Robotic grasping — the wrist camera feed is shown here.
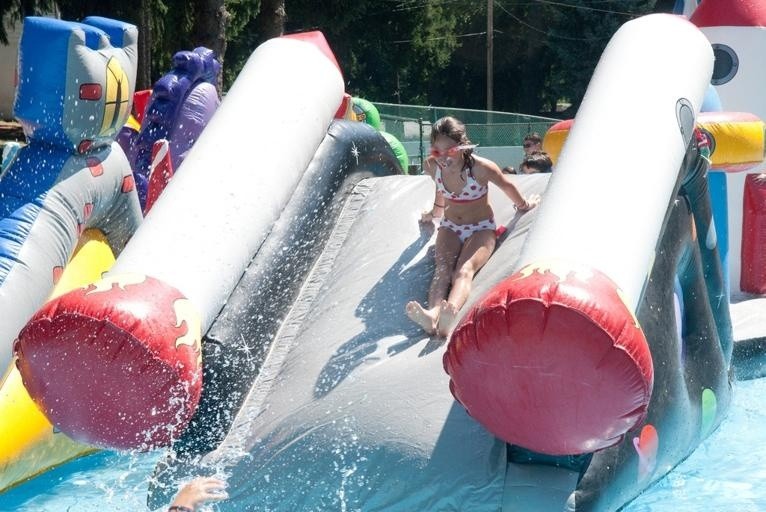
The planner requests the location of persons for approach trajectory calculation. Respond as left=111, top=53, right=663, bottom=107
left=404, top=115, right=540, bottom=339
left=518, top=152, right=553, bottom=174
left=523, top=131, right=542, bottom=154
left=164, top=475, right=228, bottom=512
left=501, top=165, right=517, bottom=174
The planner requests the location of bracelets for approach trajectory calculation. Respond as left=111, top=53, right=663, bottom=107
left=430, top=210, right=441, bottom=219
left=512, top=200, right=526, bottom=207
left=434, top=202, right=444, bottom=209
left=167, top=504, right=196, bottom=512
left=519, top=203, right=529, bottom=211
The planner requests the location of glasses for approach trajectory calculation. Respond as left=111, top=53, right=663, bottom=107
left=523, top=143, right=532, bottom=148
left=428, top=145, right=462, bottom=160
